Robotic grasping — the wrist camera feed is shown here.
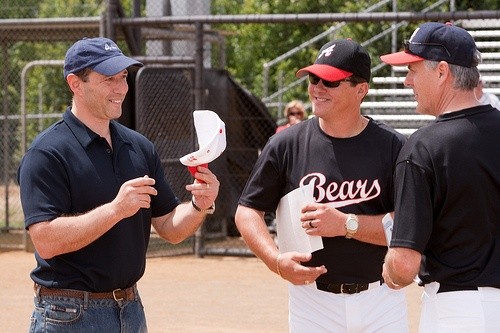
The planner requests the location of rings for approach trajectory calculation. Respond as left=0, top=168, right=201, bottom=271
left=309, top=221, right=314, bottom=228
left=305, top=281, right=310, bottom=285
left=206, top=184, right=210, bottom=189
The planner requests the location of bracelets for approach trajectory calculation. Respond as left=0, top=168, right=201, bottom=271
left=276, top=253, right=283, bottom=279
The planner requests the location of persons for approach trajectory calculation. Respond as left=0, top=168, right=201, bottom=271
left=20, top=37, right=220, bottom=333
left=235, top=40, right=408, bottom=333
left=275, top=100, right=306, bottom=133
left=382, top=22, right=500, bottom=333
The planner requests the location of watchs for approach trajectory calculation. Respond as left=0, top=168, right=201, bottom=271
left=344, top=214, right=359, bottom=238
left=192, top=198, right=215, bottom=214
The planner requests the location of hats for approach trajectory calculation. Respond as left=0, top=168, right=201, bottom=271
left=297, top=37, right=371, bottom=82
left=64, top=37, right=144, bottom=79
left=380, top=23, right=476, bottom=68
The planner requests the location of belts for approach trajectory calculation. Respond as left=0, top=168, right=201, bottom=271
left=34, top=283, right=136, bottom=302
left=316, top=281, right=368, bottom=295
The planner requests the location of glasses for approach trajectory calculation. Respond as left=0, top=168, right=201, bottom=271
left=402, top=39, right=451, bottom=57
left=309, top=72, right=369, bottom=88
left=290, top=112, right=301, bottom=115
